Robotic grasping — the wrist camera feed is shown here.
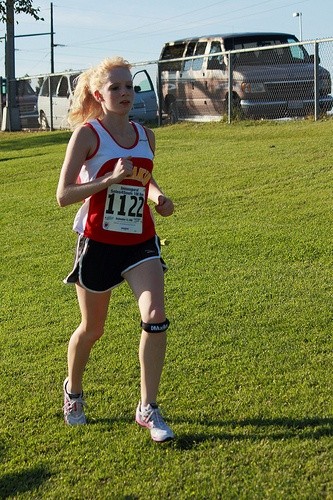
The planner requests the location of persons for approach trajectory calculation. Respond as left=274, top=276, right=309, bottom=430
left=55, top=57, right=178, bottom=442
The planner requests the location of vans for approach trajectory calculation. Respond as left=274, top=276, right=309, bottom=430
left=158, top=33, right=333, bottom=116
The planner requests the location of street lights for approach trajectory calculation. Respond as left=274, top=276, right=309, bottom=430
left=292, top=12, right=304, bottom=60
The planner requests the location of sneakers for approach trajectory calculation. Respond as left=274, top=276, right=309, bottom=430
left=136, top=399, right=175, bottom=443
left=63, top=376, right=88, bottom=426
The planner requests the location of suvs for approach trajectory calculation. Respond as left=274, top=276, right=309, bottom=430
left=36, top=70, right=159, bottom=126
left=0, top=80, right=40, bottom=130
